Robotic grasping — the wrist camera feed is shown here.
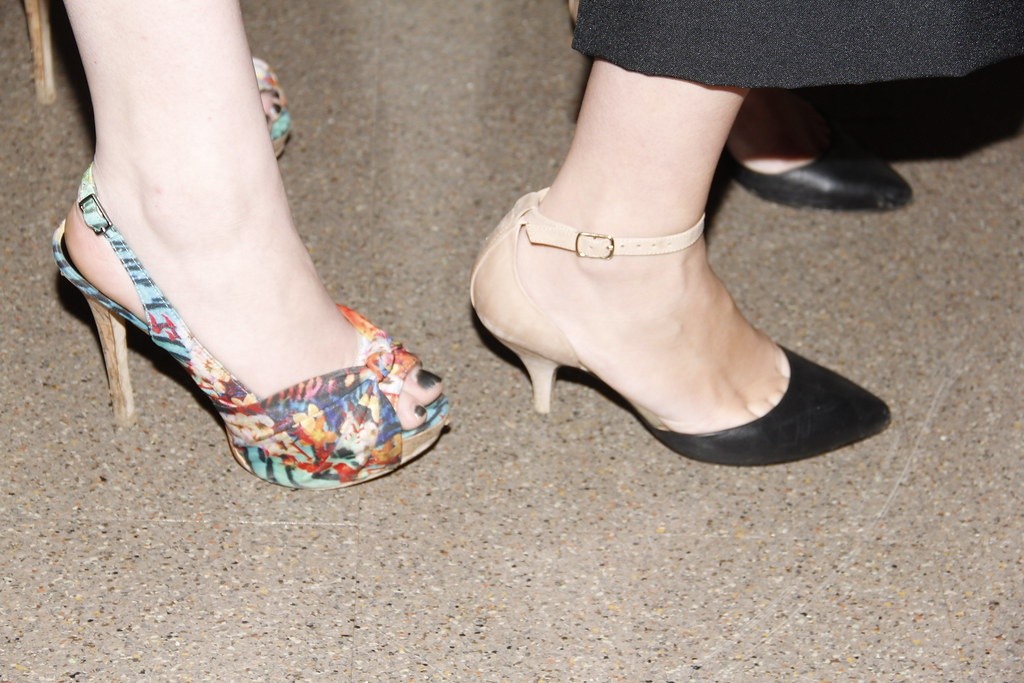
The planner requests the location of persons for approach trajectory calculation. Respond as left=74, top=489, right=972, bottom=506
left=52, top=0, right=449, bottom=491
left=469, top=0, right=1024, bottom=470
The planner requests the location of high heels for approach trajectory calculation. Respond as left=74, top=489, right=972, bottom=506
left=22, top=0, right=291, bottom=159
left=567, top=0, right=912, bottom=211
left=51, top=163, right=451, bottom=490
left=469, top=185, right=892, bottom=467
